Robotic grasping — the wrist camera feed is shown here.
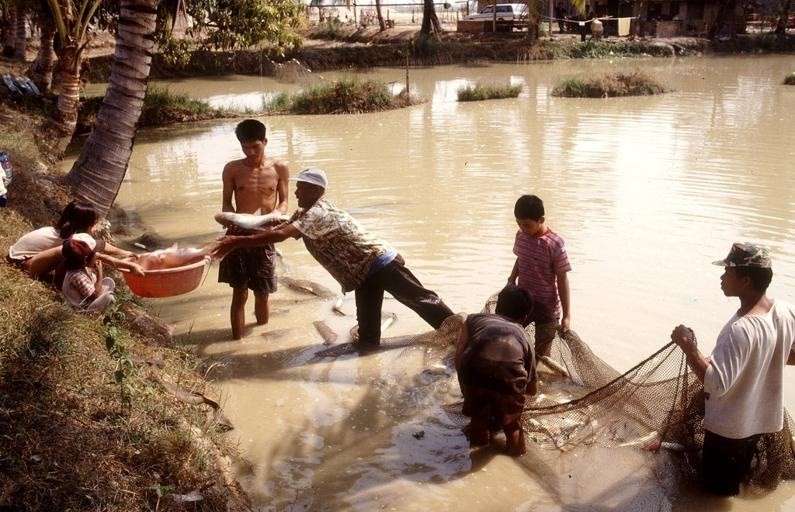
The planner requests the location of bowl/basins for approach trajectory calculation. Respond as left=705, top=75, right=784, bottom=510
left=113, top=246, right=211, bottom=300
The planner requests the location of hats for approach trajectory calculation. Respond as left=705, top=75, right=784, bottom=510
left=61, top=233, right=105, bottom=261
left=712, top=241, right=772, bottom=269
left=288, top=167, right=328, bottom=190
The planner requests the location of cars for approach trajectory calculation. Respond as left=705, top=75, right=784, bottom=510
left=462, top=3, right=534, bottom=27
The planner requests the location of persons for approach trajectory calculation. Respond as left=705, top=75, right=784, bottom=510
left=0, top=163, right=8, bottom=207
left=507, top=195, right=572, bottom=366
left=211, top=168, right=465, bottom=354
left=218, top=119, right=290, bottom=340
left=62, top=232, right=116, bottom=323
left=455, top=286, right=538, bottom=456
left=6, top=199, right=146, bottom=289
left=671, top=242, right=795, bottom=497
